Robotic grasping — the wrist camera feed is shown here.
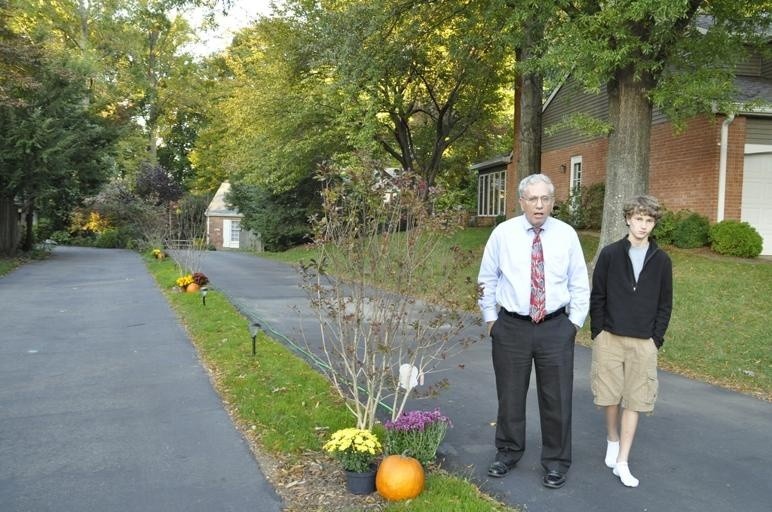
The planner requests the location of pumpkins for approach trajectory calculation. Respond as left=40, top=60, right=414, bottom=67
left=376, top=455, right=425, bottom=503
left=186, top=283, right=200, bottom=293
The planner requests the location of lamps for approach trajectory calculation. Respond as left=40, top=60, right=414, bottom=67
left=559, top=163, right=567, bottom=174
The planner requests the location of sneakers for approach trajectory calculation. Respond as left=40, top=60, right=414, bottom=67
left=613, top=461, right=639, bottom=489
left=604, top=439, right=621, bottom=469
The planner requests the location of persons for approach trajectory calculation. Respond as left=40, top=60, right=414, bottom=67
left=477, top=174, right=592, bottom=489
left=589, top=195, right=673, bottom=487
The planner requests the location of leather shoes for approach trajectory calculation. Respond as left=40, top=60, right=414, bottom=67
left=542, top=469, right=567, bottom=489
left=486, top=459, right=512, bottom=478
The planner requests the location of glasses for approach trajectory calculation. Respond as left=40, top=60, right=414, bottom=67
left=522, top=197, right=554, bottom=206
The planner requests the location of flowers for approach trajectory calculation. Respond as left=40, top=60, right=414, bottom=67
left=322, top=428, right=383, bottom=472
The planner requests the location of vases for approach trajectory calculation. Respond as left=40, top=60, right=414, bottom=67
left=345, top=464, right=377, bottom=494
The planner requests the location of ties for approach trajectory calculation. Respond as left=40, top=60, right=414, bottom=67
left=527, top=228, right=548, bottom=324
